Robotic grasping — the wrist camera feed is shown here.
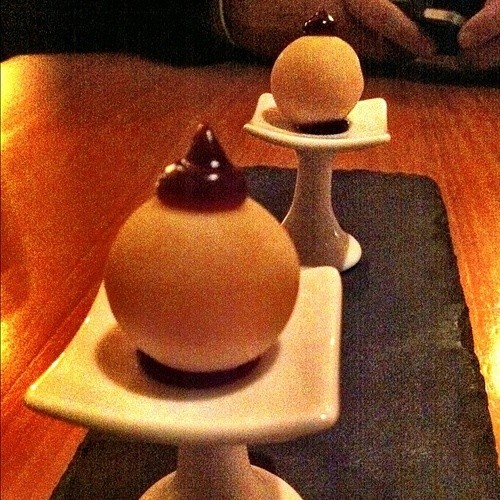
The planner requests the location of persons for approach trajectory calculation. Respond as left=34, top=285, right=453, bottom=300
left=0, top=0, right=500, bottom=82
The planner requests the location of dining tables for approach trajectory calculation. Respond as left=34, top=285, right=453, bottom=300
left=0, top=54, right=500, bottom=500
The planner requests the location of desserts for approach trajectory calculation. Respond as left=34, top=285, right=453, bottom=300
left=271, top=10, right=364, bottom=135
left=103, top=126, right=300, bottom=389
